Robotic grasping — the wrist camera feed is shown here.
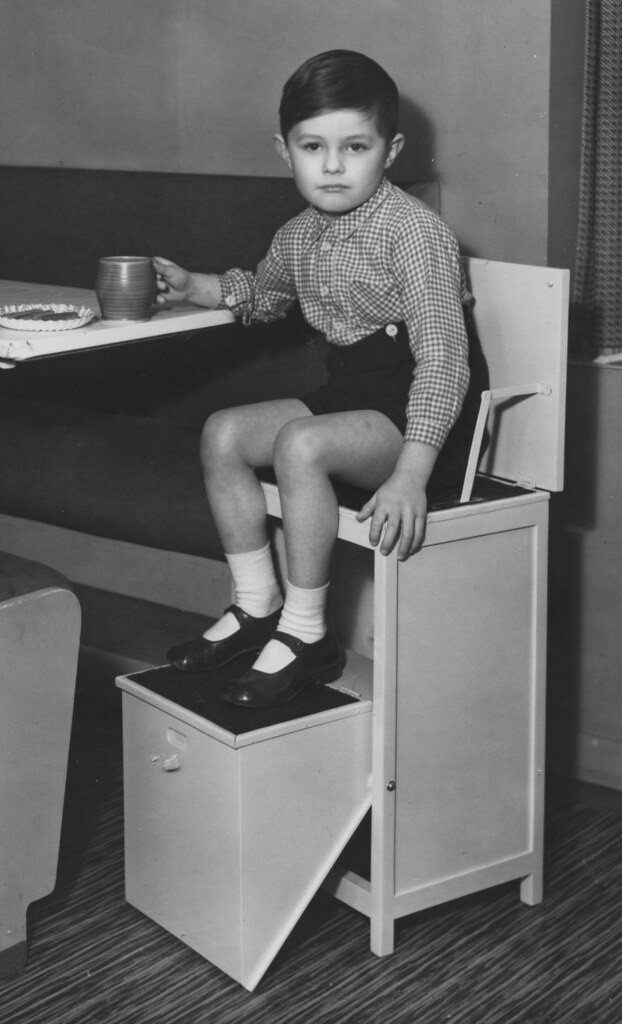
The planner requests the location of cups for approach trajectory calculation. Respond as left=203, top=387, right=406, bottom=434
left=95, top=255, right=156, bottom=323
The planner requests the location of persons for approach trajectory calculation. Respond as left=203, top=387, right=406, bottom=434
left=151, top=50, right=473, bottom=707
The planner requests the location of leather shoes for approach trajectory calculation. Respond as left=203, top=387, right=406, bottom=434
left=220, top=627, right=344, bottom=708
left=166, top=604, right=283, bottom=672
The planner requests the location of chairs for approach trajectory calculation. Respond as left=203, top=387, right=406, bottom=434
left=115, top=257, right=569, bottom=991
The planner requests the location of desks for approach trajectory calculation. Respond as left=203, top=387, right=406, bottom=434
left=0, top=277, right=240, bottom=369
